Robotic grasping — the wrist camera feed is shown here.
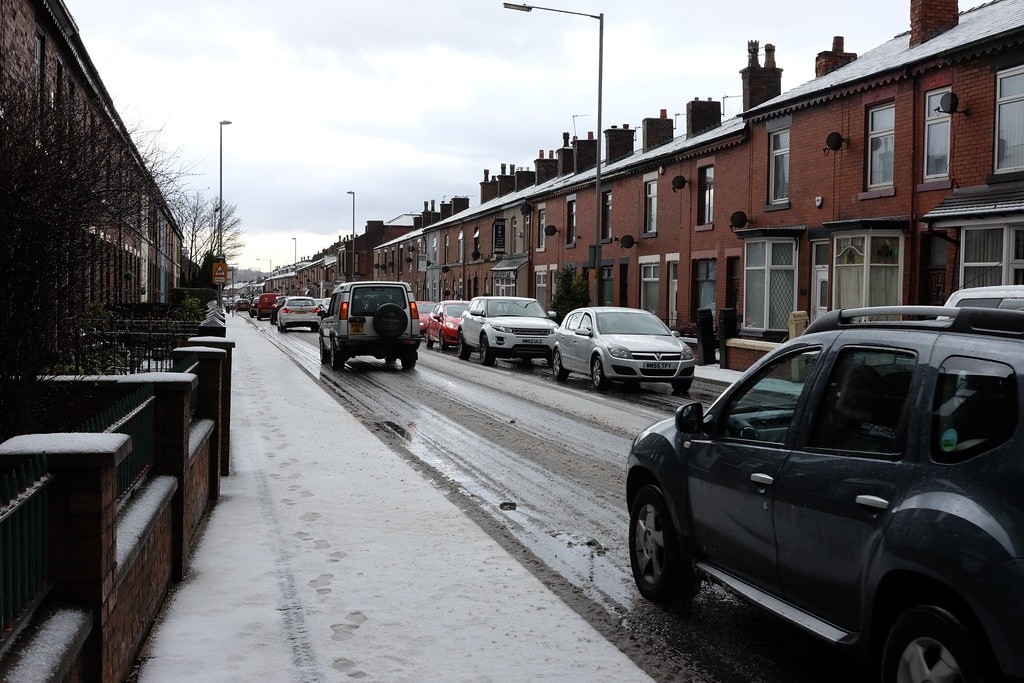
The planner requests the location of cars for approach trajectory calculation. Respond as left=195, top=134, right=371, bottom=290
left=222, top=293, right=332, bottom=325
left=416, top=301, right=438, bottom=333
left=276, top=297, right=321, bottom=334
left=551, top=307, right=696, bottom=394
left=425, top=301, right=471, bottom=351
left=457, top=296, right=559, bottom=366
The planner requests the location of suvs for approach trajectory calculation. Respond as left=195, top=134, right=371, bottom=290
left=317, top=281, right=421, bottom=372
left=623, top=306, right=1024, bottom=681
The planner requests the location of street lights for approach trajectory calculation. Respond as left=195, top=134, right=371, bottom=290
left=220, top=120, right=232, bottom=310
left=502, top=0, right=605, bottom=308
left=347, top=191, right=355, bottom=280
left=292, top=238, right=297, bottom=295
left=256, top=259, right=271, bottom=284
